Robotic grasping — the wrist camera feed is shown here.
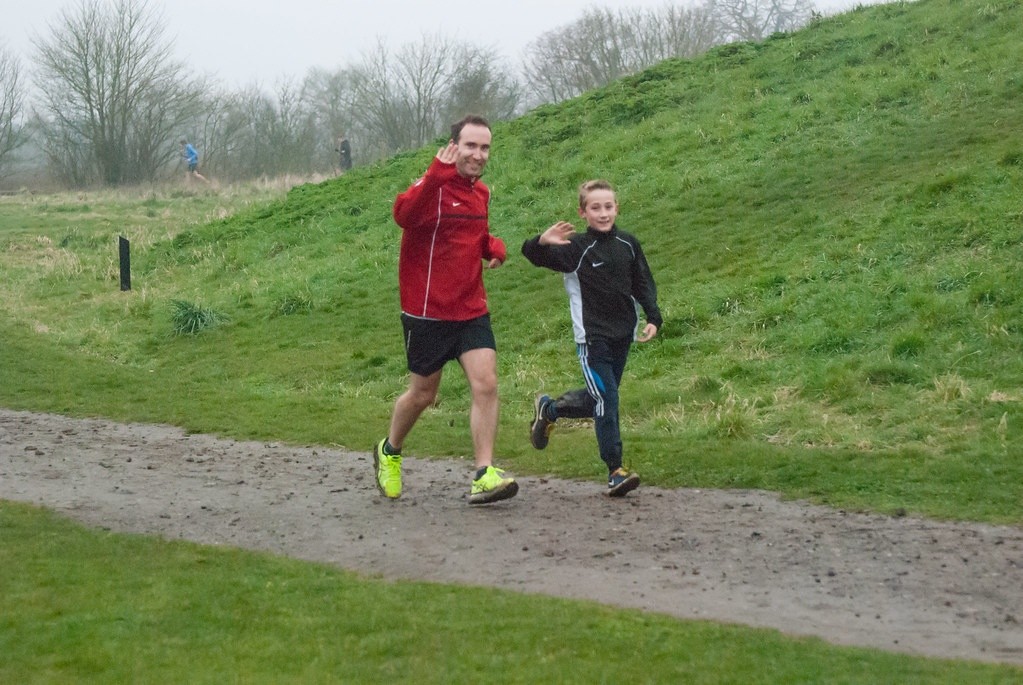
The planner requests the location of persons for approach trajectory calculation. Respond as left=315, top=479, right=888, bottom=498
left=335, top=134, right=352, bottom=172
left=374, top=116, right=518, bottom=504
left=181, top=139, right=211, bottom=189
left=521, top=180, right=661, bottom=497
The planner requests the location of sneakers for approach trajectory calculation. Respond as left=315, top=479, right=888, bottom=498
left=605, top=467, right=641, bottom=498
left=373, top=437, right=403, bottom=499
left=467, top=465, right=519, bottom=504
left=529, top=394, right=555, bottom=450
